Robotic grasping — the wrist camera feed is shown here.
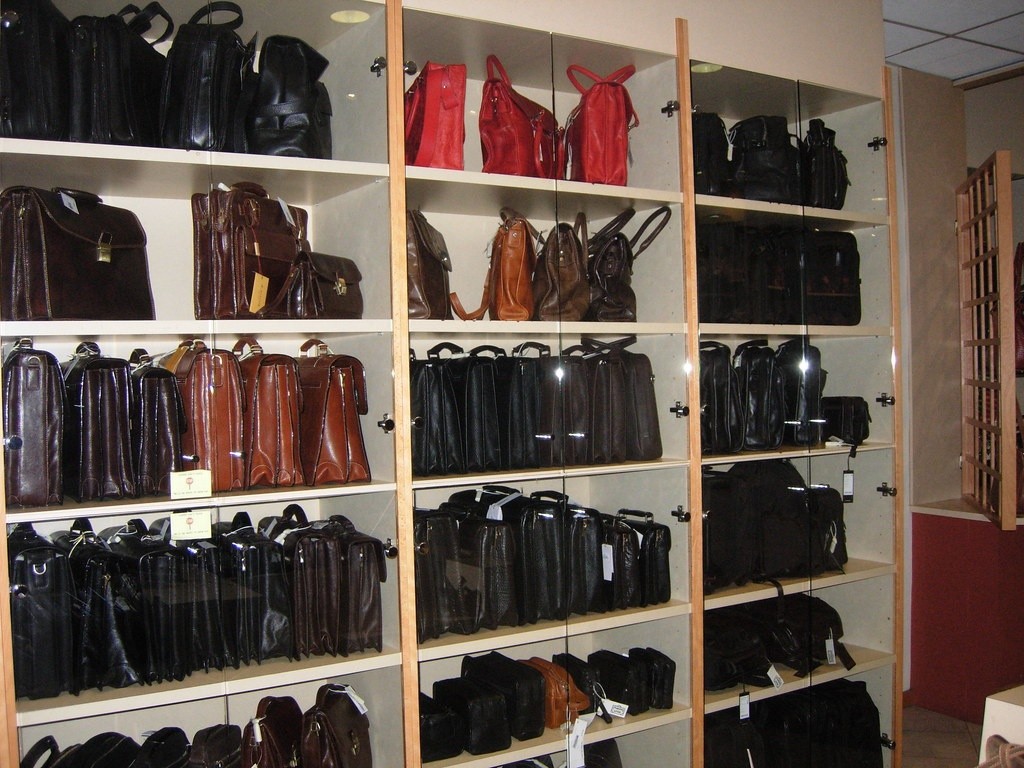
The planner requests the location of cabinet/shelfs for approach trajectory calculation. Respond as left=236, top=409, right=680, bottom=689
left=0, top=0, right=903, bottom=768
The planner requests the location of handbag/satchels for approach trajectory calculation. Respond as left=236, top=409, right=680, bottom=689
left=728, top=115, right=801, bottom=204
left=805, top=118, right=852, bottom=210
left=160, top=2, right=258, bottom=153
left=693, top=112, right=730, bottom=193
left=68, top=1, right=174, bottom=147
left=479, top=55, right=566, bottom=180
left=405, top=61, right=466, bottom=169
left=0, top=0, right=71, bottom=138
left=1, top=184, right=896, bottom=768
left=564, top=65, right=639, bottom=186
left=249, top=34, right=333, bottom=159
